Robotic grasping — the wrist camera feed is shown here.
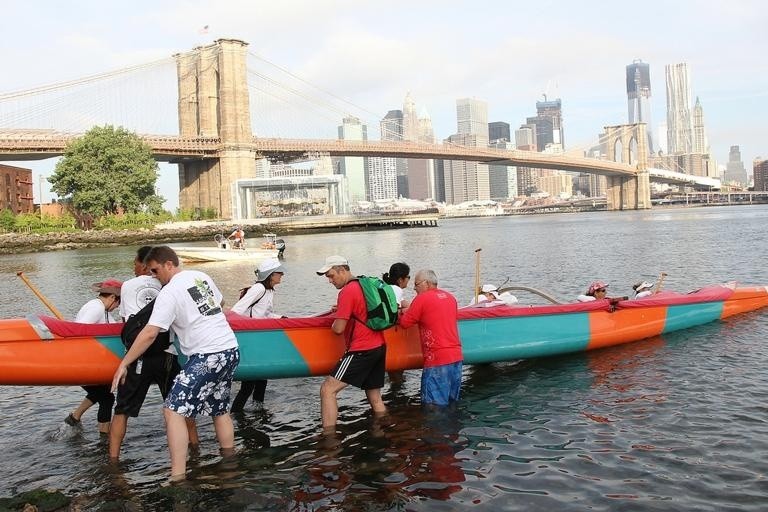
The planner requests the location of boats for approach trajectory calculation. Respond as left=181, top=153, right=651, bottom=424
left=170, top=247, right=280, bottom=261
left=0, top=284, right=768, bottom=385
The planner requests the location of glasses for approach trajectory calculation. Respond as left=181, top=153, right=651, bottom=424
left=598, top=289, right=606, bottom=292
left=414, top=279, right=426, bottom=286
left=148, top=263, right=164, bottom=275
left=405, top=276, right=411, bottom=279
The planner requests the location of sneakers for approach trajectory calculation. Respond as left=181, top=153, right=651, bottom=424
left=64, top=413, right=82, bottom=428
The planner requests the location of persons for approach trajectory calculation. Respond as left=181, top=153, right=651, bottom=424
left=111, top=245, right=242, bottom=492
left=398, top=270, right=464, bottom=405
left=468, top=283, right=499, bottom=311
left=229, top=255, right=289, bottom=412
left=109, top=245, right=199, bottom=457
left=571, top=280, right=610, bottom=304
left=380, top=261, right=411, bottom=388
left=632, top=281, right=656, bottom=301
left=316, top=256, right=388, bottom=428
left=66, top=278, right=123, bottom=436
left=226, top=225, right=245, bottom=249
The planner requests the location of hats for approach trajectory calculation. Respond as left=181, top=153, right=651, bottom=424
left=316, top=256, right=349, bottom=276
left=636, top=281, right=654, bottom=291
left=255, top=257, right=286, bottom=282
left=589, top=281, right=609, bottom=294
left=481, top=284, right=499, bottom=300
left=89, top=279, right=121, bottom=296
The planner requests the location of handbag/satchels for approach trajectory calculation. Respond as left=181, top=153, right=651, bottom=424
left=121, top=296, right=173, bottom=356
left=239, top=287, right=257, bottom=307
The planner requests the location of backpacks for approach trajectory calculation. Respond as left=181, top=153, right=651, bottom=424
left=347, top=275, right=398, bottom=330
